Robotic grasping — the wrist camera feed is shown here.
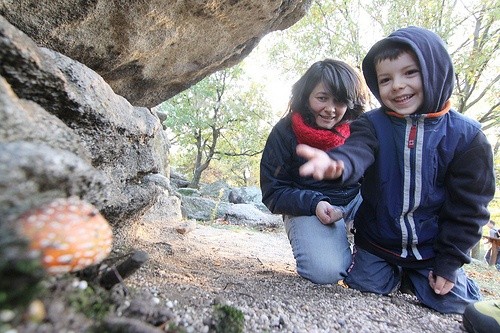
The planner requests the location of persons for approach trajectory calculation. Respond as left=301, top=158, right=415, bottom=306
left=260, top=58, right=365, bottom=284
left=486, top=220, right=500, bottom=272
left=299, top=27, right=496, bottom=315
